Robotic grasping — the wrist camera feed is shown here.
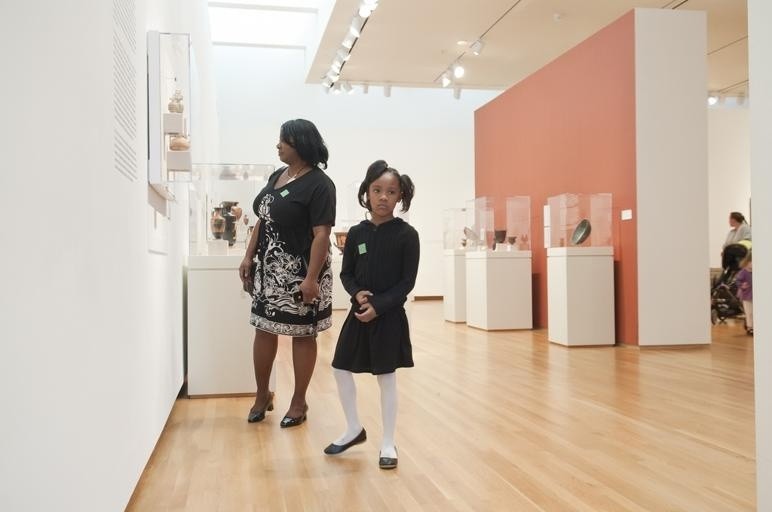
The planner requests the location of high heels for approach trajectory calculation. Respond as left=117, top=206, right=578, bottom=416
left=281, top=403, right=308, bottom=427
left=249, top=391, right=274, bottom=423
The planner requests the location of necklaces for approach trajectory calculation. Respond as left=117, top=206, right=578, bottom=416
left=287, top=166, right=303, bottom=179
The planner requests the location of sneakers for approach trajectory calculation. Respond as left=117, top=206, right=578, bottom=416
left=748, top=328, right=752, bottom=336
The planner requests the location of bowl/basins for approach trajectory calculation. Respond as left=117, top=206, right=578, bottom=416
left=571, top=219, right=591, bottom=244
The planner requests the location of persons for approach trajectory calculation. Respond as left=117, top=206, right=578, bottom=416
left=321, top=160, right=420, bottom=468
left=239, top=118, right=337, bottom=426
left=720, top=211, right=751, bottom=257
left=734, top=251, right=752, bottom=336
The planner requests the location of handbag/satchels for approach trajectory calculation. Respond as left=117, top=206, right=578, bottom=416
left=243, top=262, right=258, bottom=297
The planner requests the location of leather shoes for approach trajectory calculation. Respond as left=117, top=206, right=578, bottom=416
left=380, top=447, right=398, bottom=468
left=324, top=428, right=367, bottom=455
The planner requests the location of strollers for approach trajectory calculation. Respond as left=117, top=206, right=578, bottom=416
left=710, top=239, right=751, bottom=326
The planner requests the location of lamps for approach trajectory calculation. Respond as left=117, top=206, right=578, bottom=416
left=320, top=1, right=393, bottom=98
left=440, top=41, right=484, bottom=99
